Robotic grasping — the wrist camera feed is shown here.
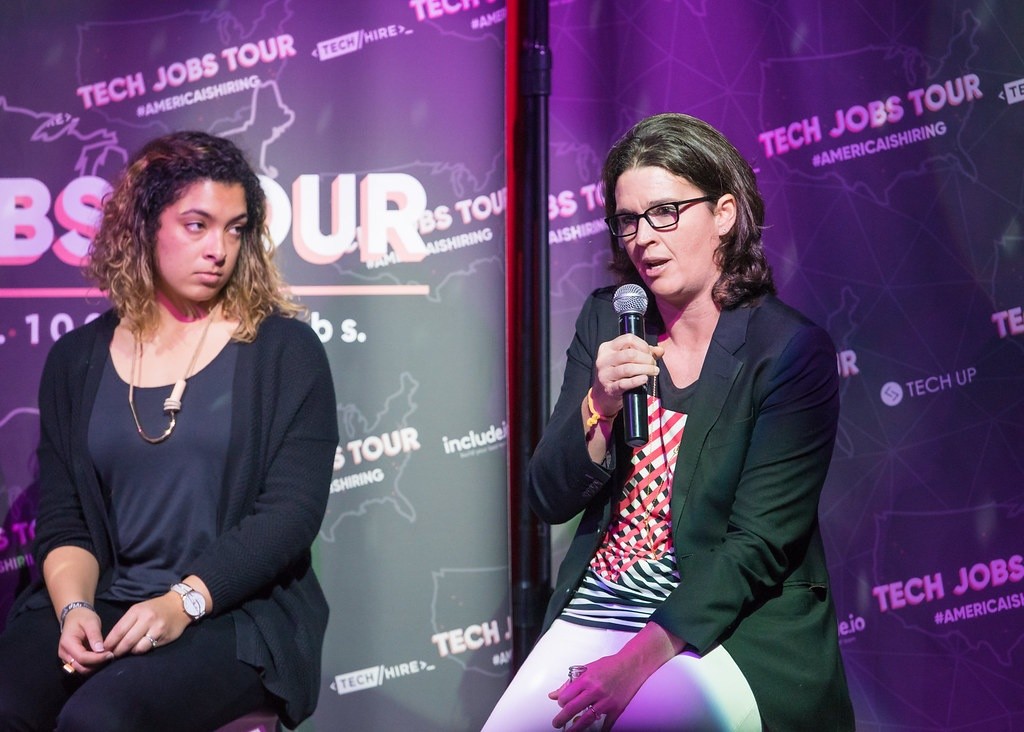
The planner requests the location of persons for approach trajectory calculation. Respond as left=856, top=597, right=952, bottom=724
left=480, top=115, right=857, bottom=732
left=0, top=132, right=338, bottom=732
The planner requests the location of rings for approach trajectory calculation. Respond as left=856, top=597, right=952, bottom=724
left=145, top=633, right=158, bottom=647
left=62, top=658, right=76, bottom=677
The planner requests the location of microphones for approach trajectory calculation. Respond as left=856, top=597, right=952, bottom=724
left=611, top=283, right=649, bottom=448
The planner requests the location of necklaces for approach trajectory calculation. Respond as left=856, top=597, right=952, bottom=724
left=128, top=305, right=219, bottom=443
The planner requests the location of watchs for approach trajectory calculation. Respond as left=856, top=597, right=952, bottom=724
left=170, top=582, right=207, bottom=624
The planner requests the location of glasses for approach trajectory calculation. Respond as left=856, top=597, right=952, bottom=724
left=604, top=195, right=714, bottom=238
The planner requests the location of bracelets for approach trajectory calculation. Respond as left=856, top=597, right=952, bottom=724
left=59, top=601, right=95, bottom=634
left=590, top=707, right=601, bottom=720
left=586, top=387, right=618, bottom=427
